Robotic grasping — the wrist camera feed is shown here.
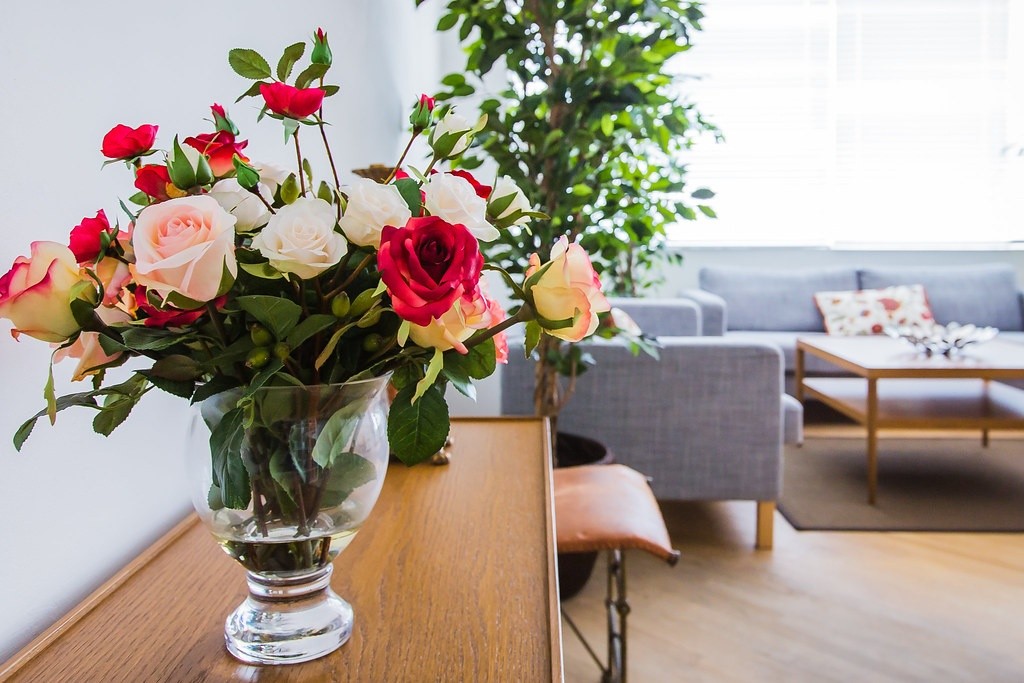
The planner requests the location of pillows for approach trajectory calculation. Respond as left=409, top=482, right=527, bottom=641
left=814, top=284, right=939, bottom=331
left=861, top=263, right=1019, bottom=330
left=700, top=266, right=859, bottom=332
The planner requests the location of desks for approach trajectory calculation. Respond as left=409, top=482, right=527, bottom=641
left=0, top=413, right=565, bottom=683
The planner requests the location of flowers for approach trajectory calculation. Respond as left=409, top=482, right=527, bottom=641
left=0, top=26, right=611, bottom=569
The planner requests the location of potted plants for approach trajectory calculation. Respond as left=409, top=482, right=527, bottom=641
left=399, top=0, right=726, bottom=608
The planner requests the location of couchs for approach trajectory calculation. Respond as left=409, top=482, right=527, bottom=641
left=507, top=245, right=1024, bottom=551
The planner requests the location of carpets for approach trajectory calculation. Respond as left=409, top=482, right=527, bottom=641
left=780, top=435, right=1024, bottom=533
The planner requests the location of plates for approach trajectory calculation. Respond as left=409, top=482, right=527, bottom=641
left=885, top=321, right=999, bottom=355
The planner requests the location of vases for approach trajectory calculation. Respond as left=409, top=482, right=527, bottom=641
left=173, top=370, right=394, bottom=666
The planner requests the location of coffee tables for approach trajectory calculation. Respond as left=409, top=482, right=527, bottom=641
left=795, top=331, right=1024, bottom=505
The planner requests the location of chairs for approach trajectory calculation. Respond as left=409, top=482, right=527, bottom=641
left=553, top=466, right=685, bottom=683
